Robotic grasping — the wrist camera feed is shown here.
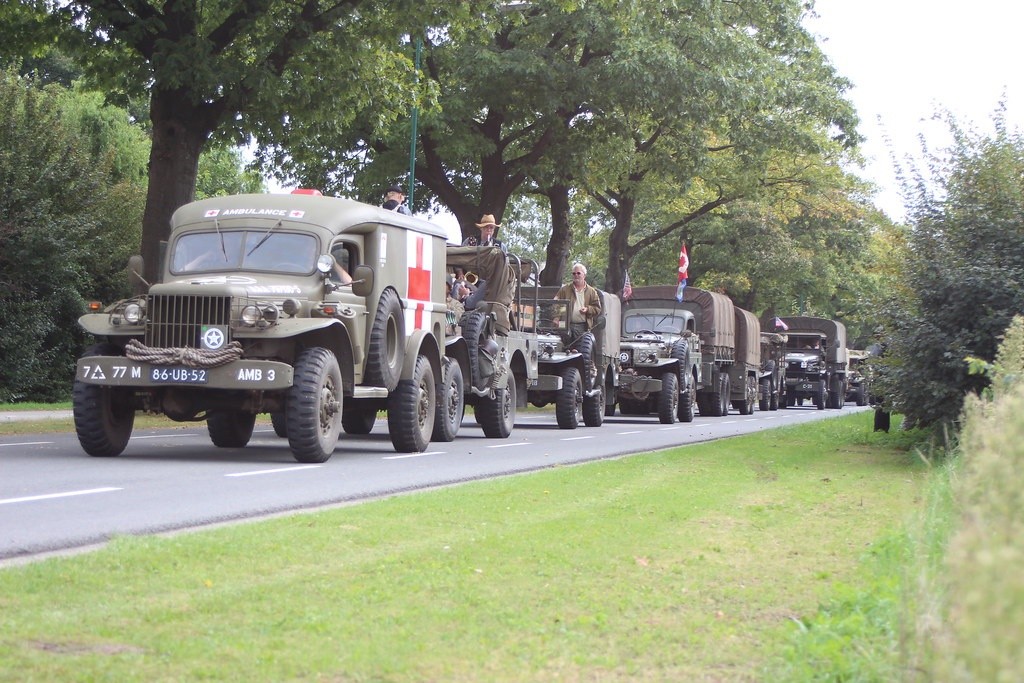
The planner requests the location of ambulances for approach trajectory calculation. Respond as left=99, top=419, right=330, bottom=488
left=73, top=189, right=451, bottom=465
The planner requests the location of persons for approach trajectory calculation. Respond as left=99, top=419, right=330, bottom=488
left=379, top=185, right=412, bottom=216
left=445, top=273, right=465, bottom=335
left=462, top=214, right=509, bottom=264
left=553, top=263, right=601, bottom=345
left=804, top=340, right=819, bottom=349
left=329, top=255, right=352, bottom=286
left=178, top=236, right=236, bottom=271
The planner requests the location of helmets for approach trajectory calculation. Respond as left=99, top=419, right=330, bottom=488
left=446, top=272, right=452, bottom=291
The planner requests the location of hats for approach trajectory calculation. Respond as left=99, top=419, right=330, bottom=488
left=384, top=186, right=402, bottom=196
left=475, top=214, right=502, bottom=228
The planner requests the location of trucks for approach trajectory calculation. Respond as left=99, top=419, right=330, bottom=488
left=434, top=242, right=540, bottom=443
left=504, top=283, right=623, bottom=430
left=758, top=332, right=790, bottom=411
left=812, top=349, right=871, bottom=406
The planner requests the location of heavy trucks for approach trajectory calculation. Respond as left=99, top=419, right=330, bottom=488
left=765, top=315, right=849, bottom=411
left=603, top=284, right=760, bottom=425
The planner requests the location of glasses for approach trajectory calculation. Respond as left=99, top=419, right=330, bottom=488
left=572, top=272, right=581, bottom=275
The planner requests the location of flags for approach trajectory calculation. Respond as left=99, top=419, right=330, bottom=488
left=776, top=317, right=788, bottom=330
left=673, top=278, right=686, bottom=302
left=677, top=241, right=690, bottom=278
left=622, top=270, right=631, bottom=301
